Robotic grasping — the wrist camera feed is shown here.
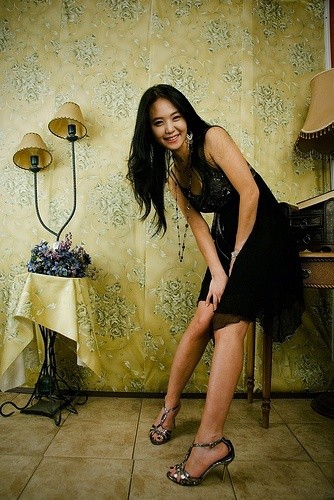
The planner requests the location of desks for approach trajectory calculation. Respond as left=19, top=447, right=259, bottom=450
left=244, top=251, right=334, bottom=428
left=0, top=271, right=102, bottom=425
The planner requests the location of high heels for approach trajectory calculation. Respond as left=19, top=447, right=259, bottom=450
left=167, top=435, right=235, bottom=486
left=150, top=401, right=182, bottom=445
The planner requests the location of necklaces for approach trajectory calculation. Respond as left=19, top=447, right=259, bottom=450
left=167, top=140, right=192, bottom=263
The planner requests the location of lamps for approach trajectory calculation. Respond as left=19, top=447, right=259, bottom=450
left=294, top=68, right=334, bottom=163
left=13, top=102, right=87, bottom=242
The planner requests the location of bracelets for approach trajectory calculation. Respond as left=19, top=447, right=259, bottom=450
left=231, top=251, right=239, bottom=257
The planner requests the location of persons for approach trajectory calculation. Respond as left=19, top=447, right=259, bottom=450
left=126, top=82, right=306, bottom=486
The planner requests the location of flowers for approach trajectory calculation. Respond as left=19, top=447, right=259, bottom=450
left=27, top=232, right=99, bottom=278
